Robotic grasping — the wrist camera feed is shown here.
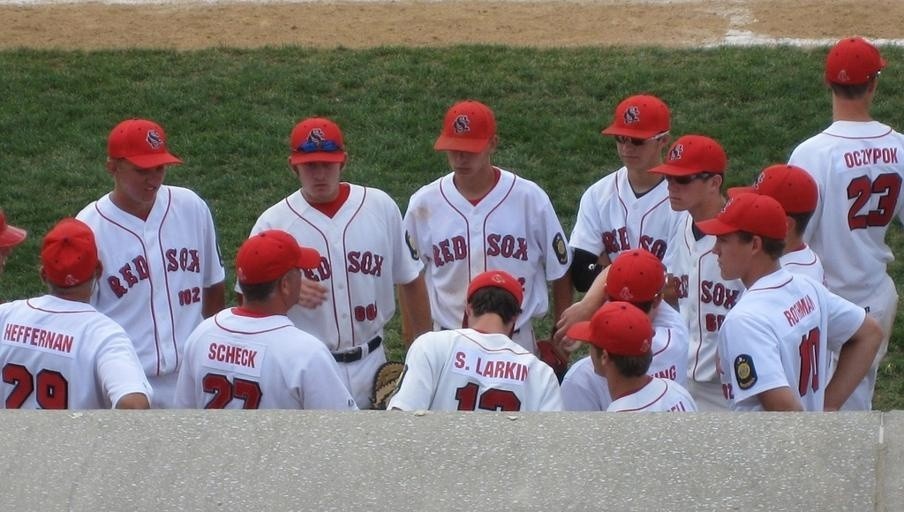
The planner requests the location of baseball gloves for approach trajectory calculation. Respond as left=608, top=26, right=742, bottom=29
left=372, top=361, right=405, bottom=410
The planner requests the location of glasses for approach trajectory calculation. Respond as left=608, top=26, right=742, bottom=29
left=661, top=171, right=712, bottom=186
left=612, top=128, right=665, bottom=147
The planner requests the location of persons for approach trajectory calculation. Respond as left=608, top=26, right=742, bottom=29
left=76, top=118, right=226, bottom=409
left=695, top=193, right=883, bottom=411
left=788, top=37, right=904, bottom=410
left=174, top=231, right=357, bottom=409
left=0, top=217, right=154, bottom=409
left=0, top=211, right=27, bottom=275
left=566, top=301, right=697, bottom=412
left=397, top=100, right=572, bottom=385
left=554, top=250, right=690, bottom=411
left=727, top=164, right=825, bottom=284
left=233, top=115, right=432, bottom=409
left=386, top=272, right=564, bottom=412
left=646, top=133, right=745, bottom=412
left=568, top=94, right=687, bottom=327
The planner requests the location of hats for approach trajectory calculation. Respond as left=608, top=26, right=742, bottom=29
left=692, top=190, right=789, bottom=243
left=107, top=118, right=183, bottom=173
left=430, top=99, right=499, bottom=156
left=607, top=247, right=670, bottom=308
left=599, top=91, right=673, bottom=141
left=38, top=218, right=100, bottom=288
left=236, top=230, right=321, bottom=282
left=647, top=132, right=728, bottom=179
left=1, top=210, right=27, bottom=250
left=288, top=116, right=350, bottom=171
left=459, top=270, right=524, bottom=337
left=568, top=298, right=656, bottom=357
left=823, top=34, right=886, bottom=90
left=728, top=165, right=821, bottom=216
